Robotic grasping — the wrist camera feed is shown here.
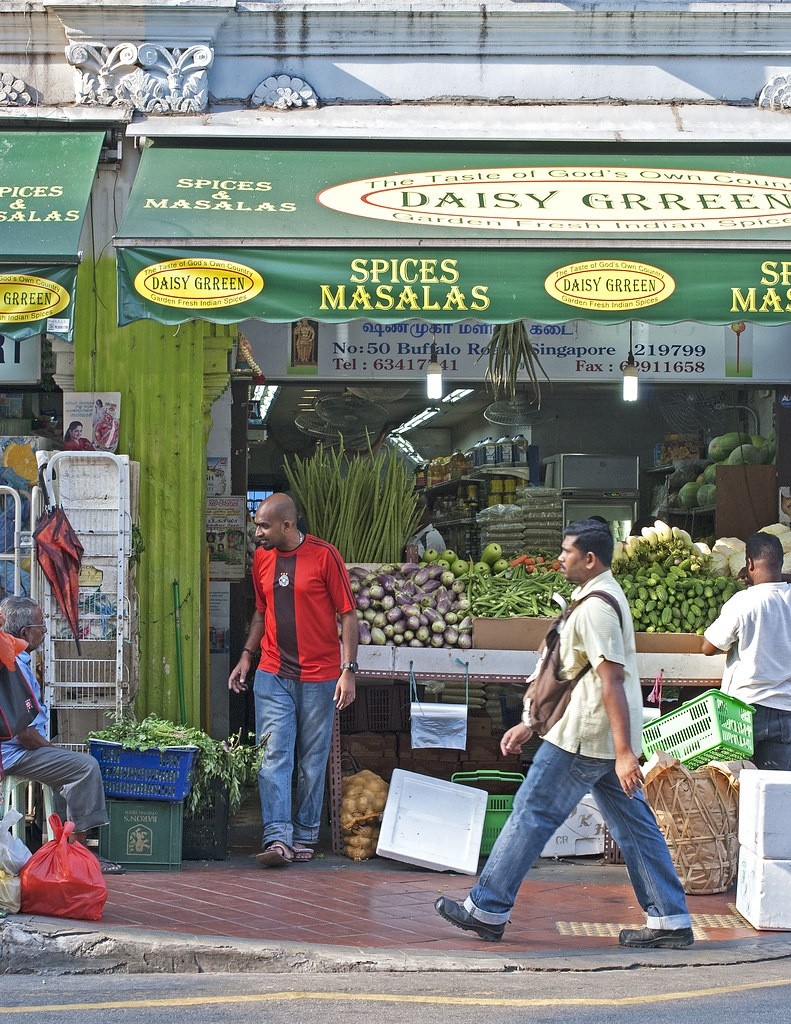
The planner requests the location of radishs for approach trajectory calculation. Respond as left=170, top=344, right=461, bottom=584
left=612, top=521, right=712, bottom=562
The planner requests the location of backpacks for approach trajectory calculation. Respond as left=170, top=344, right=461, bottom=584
left=522, top=590, right=622, bottom=737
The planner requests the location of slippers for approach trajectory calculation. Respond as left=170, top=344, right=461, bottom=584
left=256, top=845, right=293, bottom=864
left=292, top=846, right=315, bottom=861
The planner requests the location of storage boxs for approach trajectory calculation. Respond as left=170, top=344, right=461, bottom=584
left=89, top=739, right=231, bottom=870
left=341, top=614, right=791, bottom=933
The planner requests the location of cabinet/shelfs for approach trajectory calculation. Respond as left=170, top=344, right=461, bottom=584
left=415, top=461, right=532, bottom=558
left=47, top=449, right=140, bottom=846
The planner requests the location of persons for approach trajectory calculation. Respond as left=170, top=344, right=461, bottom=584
left=64, top=421, right=96, bottom=451
left=702, top=531, right=791, bottom=771
left=0, top=595, right=127, bottom=875
left=435, top=515, right=694, bottom=950
left=228, top=493, right=358, bottom=867
left=94, top=399, right=119, bottom=451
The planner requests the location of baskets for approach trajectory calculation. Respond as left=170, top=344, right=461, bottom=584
left=452, top=769, right=526, bottom=856
left=640, top=688, right=756, bottom=770
left=640, top=770, right=739, bottom=895
left=90, top=741, right=200, bottom=800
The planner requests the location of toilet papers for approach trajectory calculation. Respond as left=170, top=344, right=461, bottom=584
left=643, top=707, right=661, bottom=723
left=608, top=520, right=619, bottom=538
left=618, top=520, right=632, bottom=541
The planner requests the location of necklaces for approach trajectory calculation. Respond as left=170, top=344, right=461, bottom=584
left=297, top=530, right=303, bottom=544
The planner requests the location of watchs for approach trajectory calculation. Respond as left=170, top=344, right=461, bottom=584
left=343, top=660, right=358, bottom=673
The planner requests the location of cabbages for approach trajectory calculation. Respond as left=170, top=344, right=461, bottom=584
left=694, top=523, right=791, bottom=579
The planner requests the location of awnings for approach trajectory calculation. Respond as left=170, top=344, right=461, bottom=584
left=0, top=132, right=106, bottom=346
left=109, top=149, right=791, bottom=327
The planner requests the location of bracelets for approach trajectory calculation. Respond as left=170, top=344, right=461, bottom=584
left=240, top=648, right=256, bottom=658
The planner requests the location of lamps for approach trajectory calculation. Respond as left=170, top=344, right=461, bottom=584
left=387, top=389, right=475, bottom=464
left=248, top=386, right=282, bottom=426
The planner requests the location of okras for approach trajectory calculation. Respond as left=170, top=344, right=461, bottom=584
left=457, top=546, right=579, bottom=619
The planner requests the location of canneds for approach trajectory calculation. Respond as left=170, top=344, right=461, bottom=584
left=457, top=474, right=529, bottom=507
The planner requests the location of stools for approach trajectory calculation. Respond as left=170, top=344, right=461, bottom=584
left=1, top=776, right=58, bottom=853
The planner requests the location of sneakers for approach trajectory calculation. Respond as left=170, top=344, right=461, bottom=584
left=435, top=897, right=506, bottom=941
left=619, top=927, right=694, bottom=948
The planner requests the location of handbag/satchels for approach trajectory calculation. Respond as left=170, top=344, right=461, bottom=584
left=0, top=659, right=41, bottom=742
left=0, top=870, right=21, bottom=915
left=18, top=813, right=107, bottom=921
left=0, top=807, right=33, bottom=876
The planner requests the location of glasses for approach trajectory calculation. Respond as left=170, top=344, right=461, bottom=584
left=25, top=621, right=46, bottom=629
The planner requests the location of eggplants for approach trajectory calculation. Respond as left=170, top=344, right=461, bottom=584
left=347, top=559, right=472, bottom=648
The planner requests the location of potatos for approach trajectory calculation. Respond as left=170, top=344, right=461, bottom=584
left=340, top=770, right=388, bottom=860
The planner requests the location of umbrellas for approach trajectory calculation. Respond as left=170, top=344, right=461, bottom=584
left=32, top=461, right=86, bottom=656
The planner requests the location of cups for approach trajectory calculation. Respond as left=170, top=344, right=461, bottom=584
left=93, top=420, right=119, bottom=454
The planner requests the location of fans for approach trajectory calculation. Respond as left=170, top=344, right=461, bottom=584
left=656, top=386, right=759, bottom=448
left=483, top=383, right=557, bottom=427
left=294, top=382, right=412, bottom=443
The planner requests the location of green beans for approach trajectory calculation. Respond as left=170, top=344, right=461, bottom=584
left=85, top=710, right=271, bottom=818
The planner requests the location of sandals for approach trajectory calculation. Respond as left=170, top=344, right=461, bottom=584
left=91, top=851, right=126, bottom=874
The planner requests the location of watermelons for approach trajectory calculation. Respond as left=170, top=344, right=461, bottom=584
left=668, top=432, right=776, bottom=509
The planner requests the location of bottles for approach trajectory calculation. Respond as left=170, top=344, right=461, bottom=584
left=512, top=434, right=529, bottom=467
left=494, top=435, right=514, bottom=468
left=444, top=449, right=466, bottom=482
left=464, top=441, right=485, bottom=471
left=483, top=437, right=496, bottom=468
left=416, top=456, right=449, bottom=486
left=429, top=485, right=487, bottom=521
